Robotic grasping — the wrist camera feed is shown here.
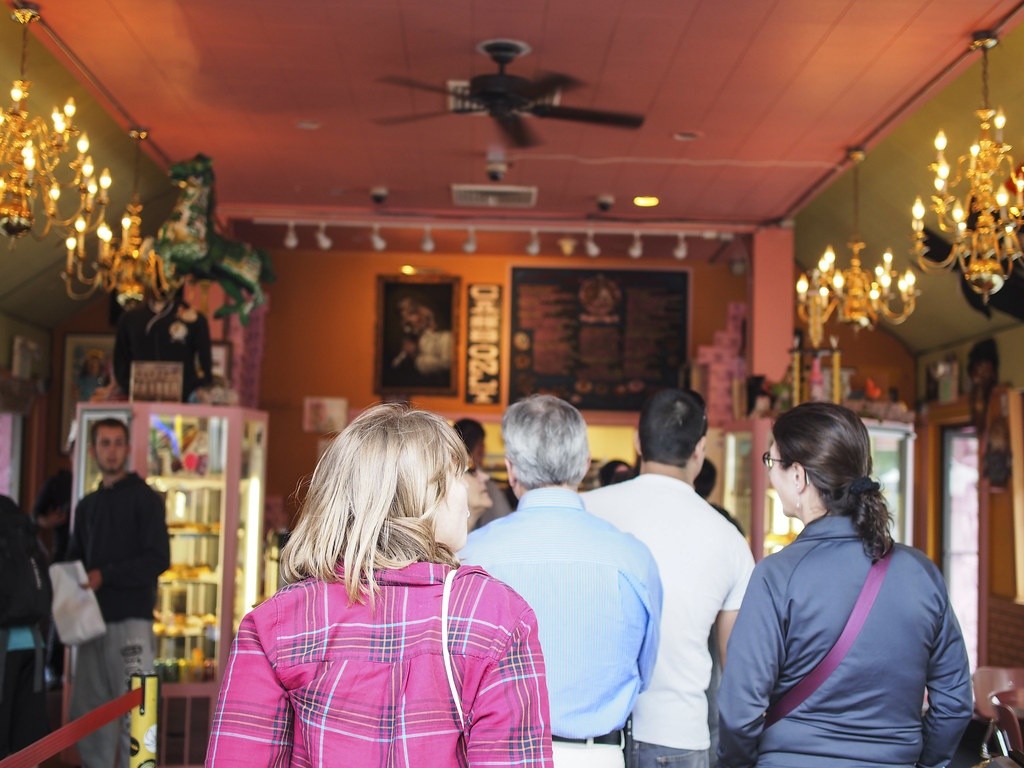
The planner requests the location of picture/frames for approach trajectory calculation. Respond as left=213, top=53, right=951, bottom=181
left=59, top=332, right=126, bottom=446
left=371, top=272, right=462, bottom=393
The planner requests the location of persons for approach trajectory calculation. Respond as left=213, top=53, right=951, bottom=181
left=452, top=395, right=663, bottom=767
left=0, top=494, right=34, bottom=750
left=454, top=418, right=512, bottom=534
left=578, top=386, right=757, bottom=768
left=66, top=416, right=171, bottom=767
left=204, top=402, right=553, bottom=768
left=599, top=457, right=746, bottom=536
left=34, top=470, right=72, bottom=689
left=717, top=402, right=974, bottom=768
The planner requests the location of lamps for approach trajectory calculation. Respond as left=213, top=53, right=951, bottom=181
left=797, top=145, right=922, bottom=348
left=907, top=27, right=1024, bottom=302
left=0, top=0, right=196, bottom=304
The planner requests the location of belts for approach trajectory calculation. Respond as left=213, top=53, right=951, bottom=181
left=550, top=729, right=622, bottom=745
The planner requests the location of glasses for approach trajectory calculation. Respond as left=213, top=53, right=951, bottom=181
left=762, top=451, right=811, bottom=485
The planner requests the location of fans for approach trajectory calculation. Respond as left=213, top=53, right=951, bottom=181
left=368, top=38, right=646, bottom=147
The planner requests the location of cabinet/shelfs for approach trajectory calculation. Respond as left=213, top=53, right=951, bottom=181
left=65, top=403, right=270, bottom=680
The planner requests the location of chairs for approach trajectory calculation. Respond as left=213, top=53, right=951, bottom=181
left=160, top=682, right=220, bottom=768
